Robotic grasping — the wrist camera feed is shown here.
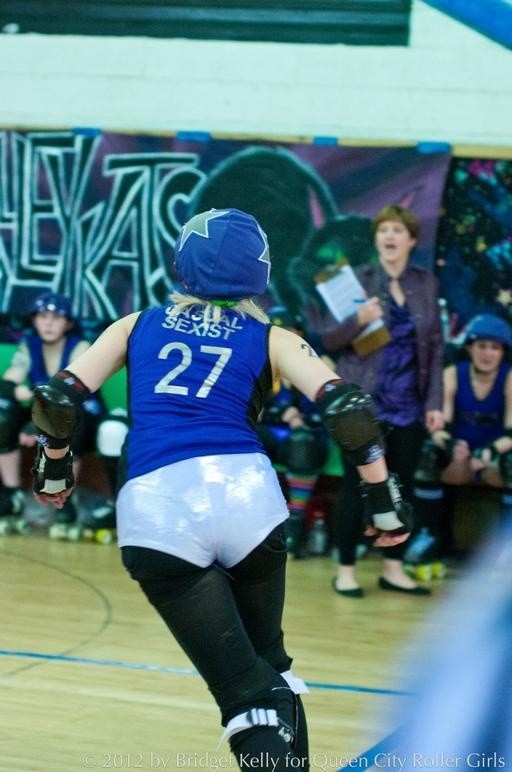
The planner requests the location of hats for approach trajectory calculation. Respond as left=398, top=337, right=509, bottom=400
left=174, top=208, right=271, bottom=300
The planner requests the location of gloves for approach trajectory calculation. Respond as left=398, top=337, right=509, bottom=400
left=287, top=430, right=325, bottom=472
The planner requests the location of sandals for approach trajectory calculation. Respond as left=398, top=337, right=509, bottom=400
left=378, top=577, right=431, bottom=595
left=332, top=577, right=363, bottom=597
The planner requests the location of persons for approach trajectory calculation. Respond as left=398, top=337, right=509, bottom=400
left=1, top=203, right=512, bottom=597
left=31, top=209, right=414, bottom=772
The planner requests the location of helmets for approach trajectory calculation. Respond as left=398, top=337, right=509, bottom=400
left=29, top=292, right=71, bottom=318
left=462, top=313, right=512, bottom=348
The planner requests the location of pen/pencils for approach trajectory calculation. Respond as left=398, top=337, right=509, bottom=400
left=353, top=298, right=368, bottom=303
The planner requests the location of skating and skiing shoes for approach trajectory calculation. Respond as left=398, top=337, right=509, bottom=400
left=0, top=488, right=118, bottom=544
left=402, top=498, right=458, bottom=585
